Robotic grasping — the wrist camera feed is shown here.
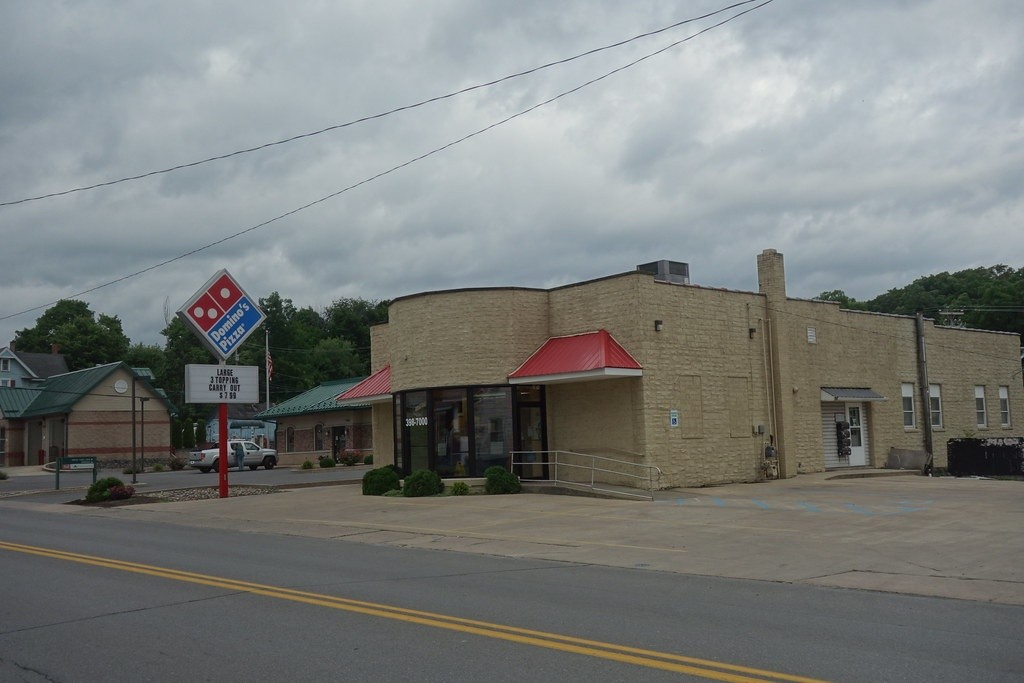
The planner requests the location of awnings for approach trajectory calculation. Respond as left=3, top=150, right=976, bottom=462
left=821, top=387, right=889, bottom=402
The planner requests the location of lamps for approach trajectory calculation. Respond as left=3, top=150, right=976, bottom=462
left=345, top=427, right=349, bottom=434
left=325, top=430, right=329, bottom=436
left=43, top=434, right=46, bottom=440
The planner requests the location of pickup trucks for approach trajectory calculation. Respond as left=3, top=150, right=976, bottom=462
left=188, top=438, right=280, bottom=472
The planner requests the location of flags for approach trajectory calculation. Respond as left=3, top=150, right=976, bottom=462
left=265, top=349, right=273, bottom=380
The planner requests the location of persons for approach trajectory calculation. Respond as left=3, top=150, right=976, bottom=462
left=338, top=434, right=345, bottom=463
